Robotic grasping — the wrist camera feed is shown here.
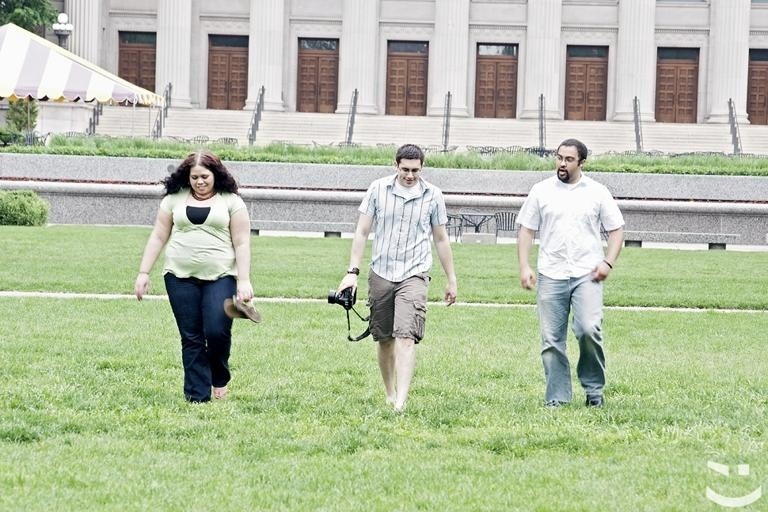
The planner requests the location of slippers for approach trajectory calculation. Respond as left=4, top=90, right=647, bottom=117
left=221, top=293, right=262, bottom=325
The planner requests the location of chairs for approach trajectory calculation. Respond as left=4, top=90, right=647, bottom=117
left=0, top=131, right=240, bottom=148
left=267, top=139, right=768, bottom=159
left=446, top=207, right=521, bottom=242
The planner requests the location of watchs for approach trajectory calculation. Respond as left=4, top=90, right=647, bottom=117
left=345, top=266, right=360, bottom=276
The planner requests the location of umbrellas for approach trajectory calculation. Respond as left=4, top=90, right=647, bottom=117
left=0, top=21, right=169, bottom=116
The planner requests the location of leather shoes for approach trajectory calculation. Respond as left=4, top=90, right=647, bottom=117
left=546, top=396, right=604, bottom=408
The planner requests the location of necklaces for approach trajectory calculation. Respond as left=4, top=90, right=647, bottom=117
left=188, top=188, right=218, bottom=201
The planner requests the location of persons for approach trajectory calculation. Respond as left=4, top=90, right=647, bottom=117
left=516, top=138, right=626, bottom=412
left=333, top=143, right=459, bottom=417
left=133, top=150, right=256, bottom=404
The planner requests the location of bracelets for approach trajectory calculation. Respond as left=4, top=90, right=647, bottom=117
left=602, top=259, right=613, bottom=270
left=137, top=270, right=149, bottom=275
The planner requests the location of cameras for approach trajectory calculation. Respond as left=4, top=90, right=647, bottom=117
left=328, top=288, right=356, bottom=310
